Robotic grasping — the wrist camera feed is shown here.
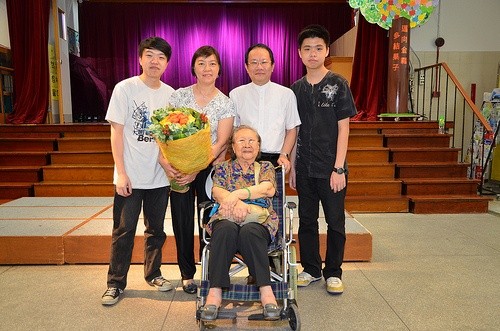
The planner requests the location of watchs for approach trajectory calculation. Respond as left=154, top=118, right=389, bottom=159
left=333, top=167, right=345, bottom=174
left=281, top=153, right=288, bottom=158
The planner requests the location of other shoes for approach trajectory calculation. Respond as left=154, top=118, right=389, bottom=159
left=263, top=303, right=280, bottom=321
left=182, top=279, right=198, bottom=293
left=200, top=302, right=222, bottom=322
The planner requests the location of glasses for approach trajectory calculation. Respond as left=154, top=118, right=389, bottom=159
left=248, top=60, right=273, bottom=68
left=235, top=138, right=261, bottom=144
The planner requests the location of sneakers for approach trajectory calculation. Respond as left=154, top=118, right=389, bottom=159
left=148, top=276, right=173, bottom=291
left=297, top=271, right=322, bottom=287
left=101, top=287, right=123, bottom=305
left=326, top=277, right=344, bottom=293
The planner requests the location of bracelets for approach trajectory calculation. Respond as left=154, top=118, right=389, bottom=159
left=244, top=187, right=251, bottom=199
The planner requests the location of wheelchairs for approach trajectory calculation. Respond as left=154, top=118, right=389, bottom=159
left=195, top=162, right=303, bottom=331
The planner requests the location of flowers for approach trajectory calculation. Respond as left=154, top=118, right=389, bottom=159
left=149, top=107, right=210, bottom=147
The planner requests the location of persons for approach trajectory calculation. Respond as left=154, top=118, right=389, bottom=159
left=158, top=46, right=236, bottom=294
left=200, top=125, right=281, bottom=322
left=100, top=37, right=176, bottom=304
left=228, top=43, right=301, bottom=285
left=288, top=27, right=357, bottom=293
